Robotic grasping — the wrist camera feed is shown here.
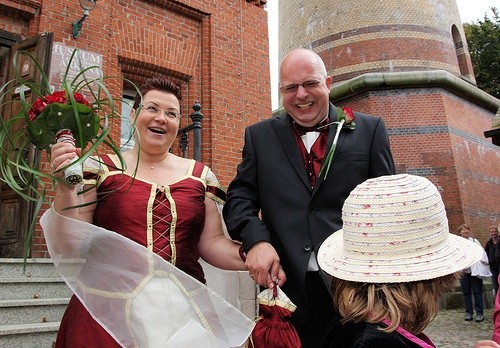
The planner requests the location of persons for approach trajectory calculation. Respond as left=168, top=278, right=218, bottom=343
left=317, top=172, right=486, bottom=348
left=484, top=225, right=500, bottom=306
left=471, top=270, right=500, bottom=348
left=221, top=47, right=396, bottom=348
left=49, top=78, right=286, bottom=348
left=456, top=224, right=485, bottom=322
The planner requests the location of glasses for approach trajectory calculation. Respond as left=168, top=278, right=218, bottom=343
left=134, top=104, right=183, bottom=122
left=281, top=78, right=325, bottom=94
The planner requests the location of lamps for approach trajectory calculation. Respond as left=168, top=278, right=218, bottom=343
left=72, top=0, right=97, bottom=39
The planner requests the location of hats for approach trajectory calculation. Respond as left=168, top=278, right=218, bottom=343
left=317, top=174, right=484, bottom=283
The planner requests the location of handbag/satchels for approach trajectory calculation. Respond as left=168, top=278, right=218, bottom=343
left=239, top=244, right=302, bottom=348
left=482, top=265, right=493, bottom=284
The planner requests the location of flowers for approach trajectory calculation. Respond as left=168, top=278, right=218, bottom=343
left=317, top=106, right=357, bottom=180
left=0, top=48, right=143, bottom=275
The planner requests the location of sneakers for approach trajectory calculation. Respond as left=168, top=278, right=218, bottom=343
left=476, top=314, right=484, bottom=322
left=465, top=314, right=473, bottom=321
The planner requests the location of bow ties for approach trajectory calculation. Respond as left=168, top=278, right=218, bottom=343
left=292, top=117, right=330, bottom=137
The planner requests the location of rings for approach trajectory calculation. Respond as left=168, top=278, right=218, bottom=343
left=248, top=273, right=255, bottom=277
left=51, top=162, right=56, bottom=168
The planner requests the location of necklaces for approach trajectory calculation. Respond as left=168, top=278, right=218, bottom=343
left=131, top=150, right=170, bottom=169
